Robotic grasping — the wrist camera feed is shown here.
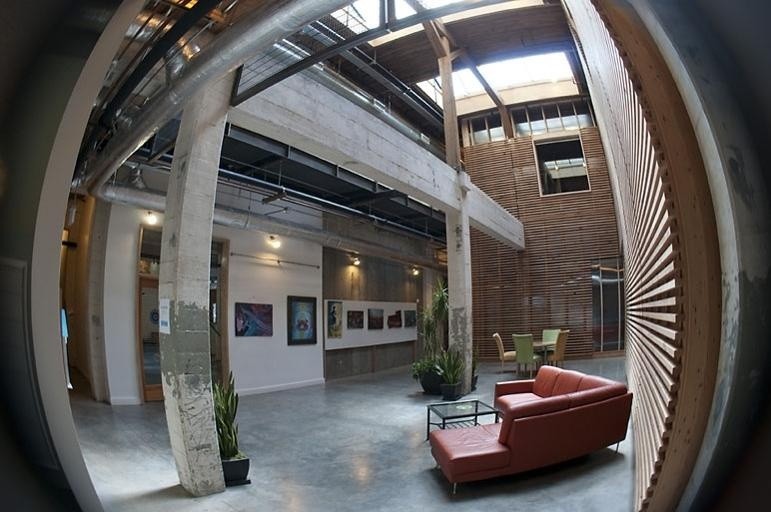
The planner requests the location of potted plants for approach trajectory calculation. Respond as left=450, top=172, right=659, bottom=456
left=407, top=352, right=465, bottom=401
left=211, top=371, right=252, bottom=488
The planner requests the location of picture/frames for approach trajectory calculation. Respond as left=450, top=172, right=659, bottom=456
left=286, top=295, right=317, bottom=345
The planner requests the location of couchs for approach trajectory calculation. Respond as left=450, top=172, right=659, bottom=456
left=427, top=364, right=634, bottom=495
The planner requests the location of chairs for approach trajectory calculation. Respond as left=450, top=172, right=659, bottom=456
left=492, top=328, right=571, bottom=380
